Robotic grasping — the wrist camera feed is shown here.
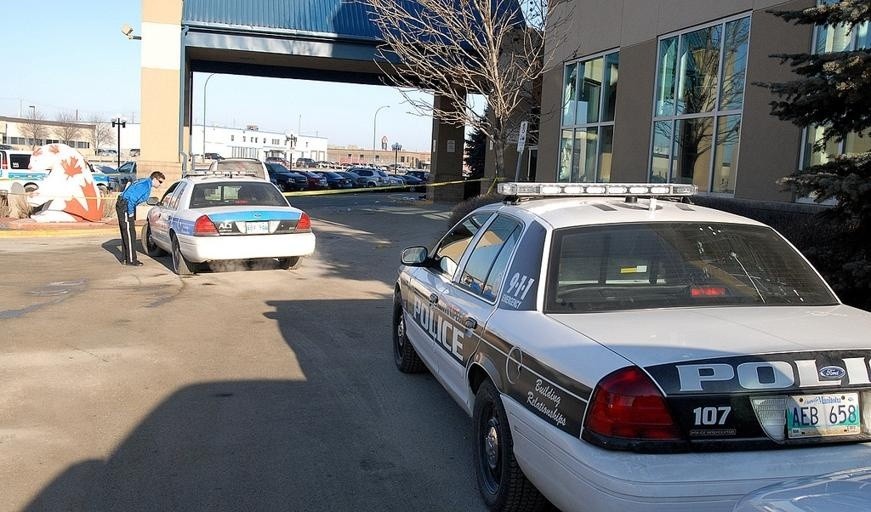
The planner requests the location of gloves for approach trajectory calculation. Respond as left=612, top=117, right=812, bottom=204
left=129, top=214, right=134, bottom=222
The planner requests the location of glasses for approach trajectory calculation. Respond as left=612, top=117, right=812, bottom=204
left=157, top=179, right=162, bottom=184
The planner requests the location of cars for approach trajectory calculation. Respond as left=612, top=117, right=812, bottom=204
left=137, top=173, right=314, bottom=273
left=391, top=164, right=430, bottom=185
left=388, top=181, right=871, bottom=510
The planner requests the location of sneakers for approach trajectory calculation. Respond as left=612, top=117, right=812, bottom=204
left=128, top=261, right=143, bottom=266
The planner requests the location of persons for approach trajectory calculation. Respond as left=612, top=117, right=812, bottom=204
left=114, top=169, right=165, bottom=268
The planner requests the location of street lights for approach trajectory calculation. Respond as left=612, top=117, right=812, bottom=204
left=730, top=252, right=766, bottom=304
left=285, top=130, right=297, bottom=170
left=392, top=142, right=402, bottom=174
left=29, top=104, right=37, bottom=145
left=372, top=105, right=390, bottom=160
left=111, top=115, right=127, bottom=167
left=203, top=73, right=222, bottom=159
